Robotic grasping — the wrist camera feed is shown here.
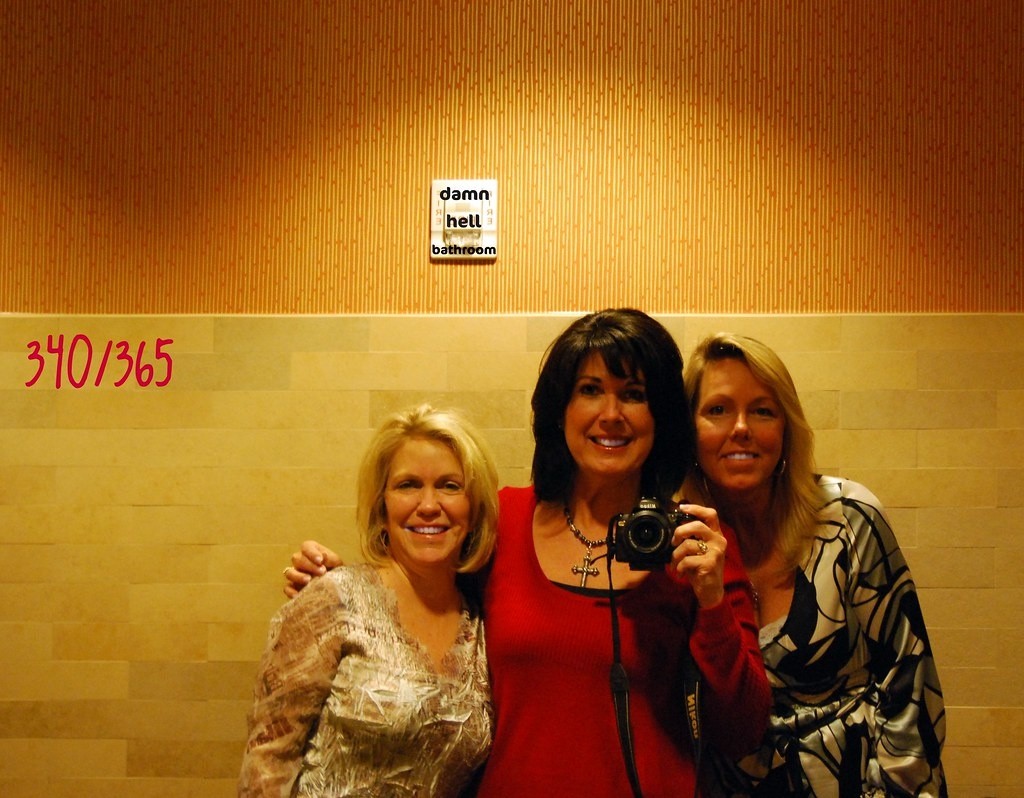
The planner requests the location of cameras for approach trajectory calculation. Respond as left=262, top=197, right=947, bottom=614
left=615, top=497, right=702, bottom=571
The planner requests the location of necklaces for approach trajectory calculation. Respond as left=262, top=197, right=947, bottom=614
left=561, top=498, right=649, bottom=593
left=716, top=500, right=781, bottom=612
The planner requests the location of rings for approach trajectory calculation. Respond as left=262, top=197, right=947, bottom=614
left=692, top=538, right=711, bottom=557
left=282, top=566, right=296, bottom=579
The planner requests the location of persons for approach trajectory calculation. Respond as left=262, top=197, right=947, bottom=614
left=279, top=307, right=771, bottom=798
left=237, top=404, right=500, bottom=798
left=683, top=329, right=948, bottom=798
